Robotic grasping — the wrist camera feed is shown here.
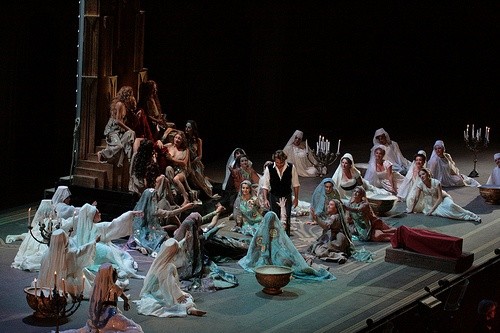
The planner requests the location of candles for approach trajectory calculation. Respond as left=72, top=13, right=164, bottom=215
left=82, top=274, right=86, bottom=291
left=317, top=135, right=330, bottom=154
left=485, top=126, right=490, bottom=142
left=472, top=124, right=474, bottom=138
left=28, top=208, right=32, bottom=226
left=464, top=124, right=470, bottom=139
left=62, top=278, right=65, bottom=293
left=34, top=277, right=37, bottom=297
left=477, top=127, right=481, bottom=140
left=338, top=139, right=342, bottom=153
left=54, top=269, right=57, bottom=288
left=306, top=139, right=308, bottom=153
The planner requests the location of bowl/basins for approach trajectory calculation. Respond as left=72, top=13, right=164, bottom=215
left=479, top=185, right=500, bottom=204
left=366, top=196, right=396, bottom=217
left=253, top=265, right=294, bottom=294
left=24, top=287, right=67, bottom=318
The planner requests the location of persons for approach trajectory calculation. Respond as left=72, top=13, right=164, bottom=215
left=49, top=263, right=146, bottom=333
left=309, top=128, right=482, bottom=265
left=98, top=79, right=239, bottom=290
left=7, top=186, right=146, bottom=301
left=486, top=153, right=500, bottom=186
left=132, top=230, right=208, bottom=319
left=283, top=130, right=327, bottom=178
left=237, top=196, right=336, bottom=282
left=222, top=147, right=311, bottom=240
